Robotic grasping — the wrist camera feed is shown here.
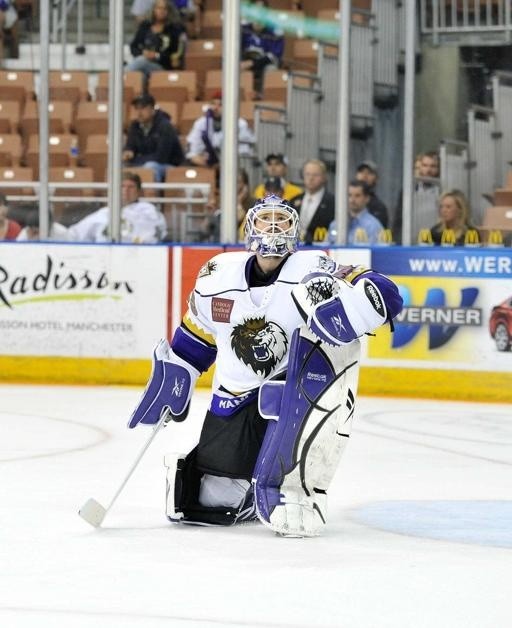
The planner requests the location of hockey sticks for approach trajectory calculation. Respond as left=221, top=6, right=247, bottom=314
left=77, top=407, right=170, bottom=527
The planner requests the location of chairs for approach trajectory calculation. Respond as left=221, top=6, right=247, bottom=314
left=1, top=0, right=512, bottom=246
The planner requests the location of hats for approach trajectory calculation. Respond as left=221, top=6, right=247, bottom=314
left=206, top=89, right=222, bottom=99
left=263, top=175, right=284, bottom=191
left=265, top=150, right=289, bottom=166
left=130, top=93, right=155, bottom=108
left=357, top=158, right=378, bottom=173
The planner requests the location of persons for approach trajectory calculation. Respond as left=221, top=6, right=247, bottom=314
left=0, top=1, right=288, bottom=197
left=170, top=194, right=403, bottom=525
left=1, top=150, right=482, bottom=245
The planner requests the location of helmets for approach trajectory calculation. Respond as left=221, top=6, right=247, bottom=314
left=241, top=194, right=301, bottom=258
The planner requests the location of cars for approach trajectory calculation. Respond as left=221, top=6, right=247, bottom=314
left=489, top=295, right=512, bottom=353
